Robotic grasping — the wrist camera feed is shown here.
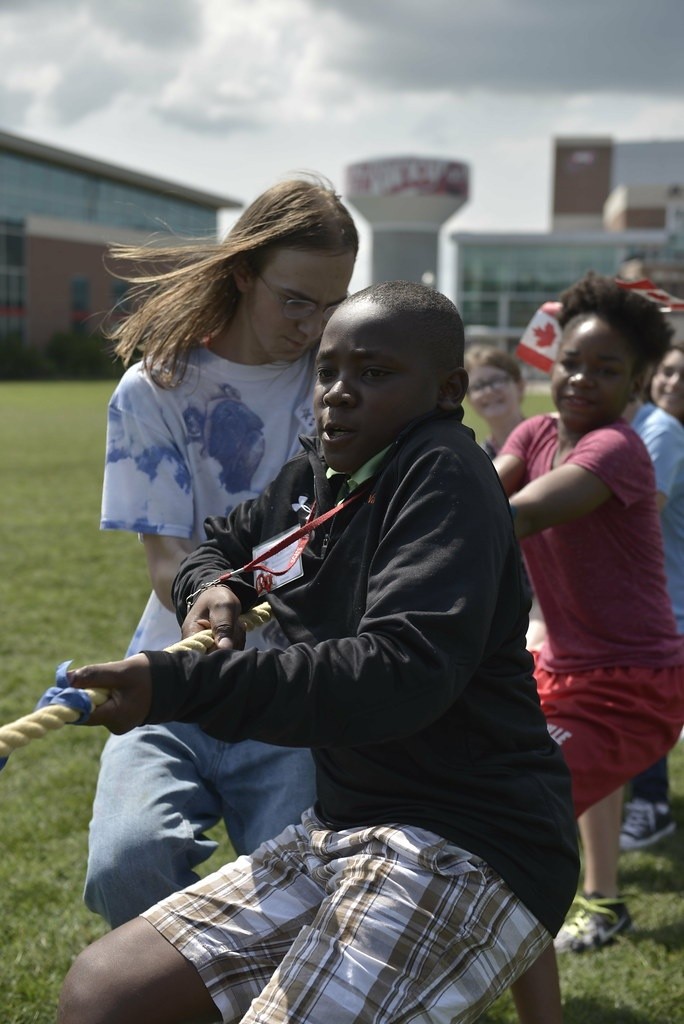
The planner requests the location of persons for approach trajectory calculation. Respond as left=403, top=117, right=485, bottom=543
left=83, top=178, right=361, bottom=933
left=465, top=279, right=684, bottom=953
left=58, top=283, right=580, bottom=1024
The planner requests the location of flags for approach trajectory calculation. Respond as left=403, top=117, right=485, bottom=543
left=517, top=280, right=684, bottom=372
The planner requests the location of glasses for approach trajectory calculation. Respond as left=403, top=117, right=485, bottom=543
left=466, top=371, right=512, bottom=398
left=255, top=275, right=339, bottom=325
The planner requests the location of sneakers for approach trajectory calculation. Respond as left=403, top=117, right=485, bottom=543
left=620, top=799, right=676, bottom=851
left=553, top=891, right=631, bottom=955
